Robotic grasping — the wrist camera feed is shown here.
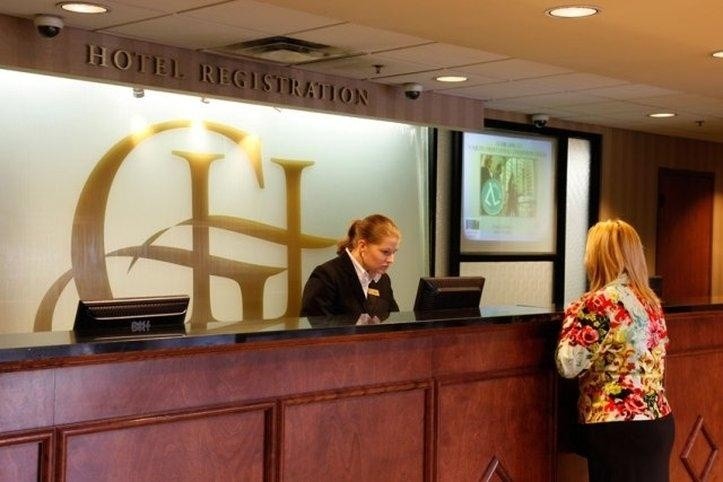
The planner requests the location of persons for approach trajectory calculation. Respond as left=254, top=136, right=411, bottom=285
left=554, top=219, right=676, bottom=482
left=301, top=214, right=400, bottom=317
left=480, top=155, right=517, bottom=217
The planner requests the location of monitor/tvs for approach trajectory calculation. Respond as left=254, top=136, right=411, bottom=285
left=413, top=277, right=485, bottom=311
left=73, top=295, right=190, bottom=331
left=649, top=276, right=663, bottom=300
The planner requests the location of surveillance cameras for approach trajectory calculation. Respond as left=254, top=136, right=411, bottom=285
left=532, top=114, right=550, bottom=128
left=404, top=84, right=423, bottom=100
left=33, top=15, right=65, bottom=38
